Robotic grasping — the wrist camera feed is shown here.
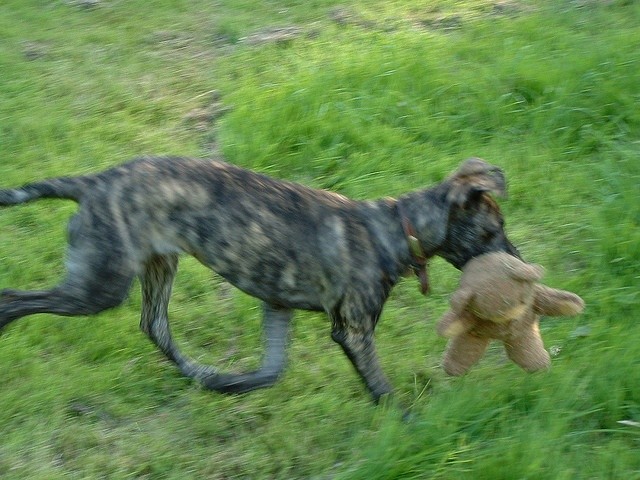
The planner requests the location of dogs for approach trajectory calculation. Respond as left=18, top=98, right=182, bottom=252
left=0, top=155, right=526, bottom=406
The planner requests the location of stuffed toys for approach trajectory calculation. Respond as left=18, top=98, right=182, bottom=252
left=436, top=252, right=586, bottom=379
